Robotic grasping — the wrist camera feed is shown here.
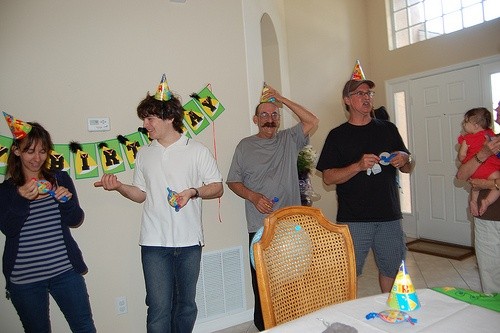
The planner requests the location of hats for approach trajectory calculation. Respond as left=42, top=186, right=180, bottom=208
left=343, top=79, right=376, bottom=98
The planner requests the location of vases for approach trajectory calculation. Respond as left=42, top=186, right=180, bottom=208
left=298, top=178, right=312, bottom=206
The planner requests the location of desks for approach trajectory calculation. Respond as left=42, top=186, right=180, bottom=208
left=257, top=287, right=500, bottom=333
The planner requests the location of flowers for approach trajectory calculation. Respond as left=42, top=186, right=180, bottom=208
left=297, top=145, right=318, bottom=176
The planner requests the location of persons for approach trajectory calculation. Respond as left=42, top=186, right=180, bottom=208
left=316, top=80, right=416, bottom=293
left=456, top=101, right=500, bottom=296
left=94, top=93, right=224, bottom=333
left=0, top=122, right=96, bottom=333
left=226, top=86, right=320, bottom=330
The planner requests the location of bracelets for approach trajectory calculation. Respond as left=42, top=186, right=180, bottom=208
left=494, top=179, right=500, bottom=190
left=190, top=188, right=199, bottom=197
left=475, top=153, right=484, bottom=164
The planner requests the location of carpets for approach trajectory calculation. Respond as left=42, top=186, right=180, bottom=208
left=405, top=239, right=474, bottom=260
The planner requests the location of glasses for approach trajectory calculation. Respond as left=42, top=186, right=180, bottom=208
left=256, top=112, right=281, bottom=121
left=348, top=90, right=376, bottom=97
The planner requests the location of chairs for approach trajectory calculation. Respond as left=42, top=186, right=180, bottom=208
left=253, top=206, right=357, bottom=330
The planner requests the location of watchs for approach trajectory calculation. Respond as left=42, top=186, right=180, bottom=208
left=408, top=154, right=412, bottom=164
